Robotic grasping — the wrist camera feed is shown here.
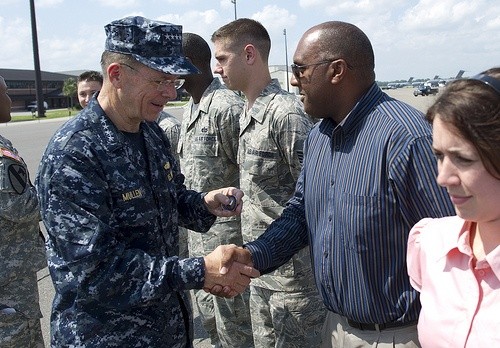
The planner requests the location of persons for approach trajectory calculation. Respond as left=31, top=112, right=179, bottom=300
left=78, top=70, right=103, bottom=109
left=406, top=67, right=500, bottom=348
left=155, top=17, right=329, bottom=348
left=38, top=16, right=261, bottom=348
left=0, top=75, right=48, bottom=348
left=203, top=20, right=457, bottom=348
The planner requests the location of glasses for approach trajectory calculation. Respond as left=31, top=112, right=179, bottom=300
left=292, top=59, right=353, bottom=77
left=118, top=63, right=185, bottom=90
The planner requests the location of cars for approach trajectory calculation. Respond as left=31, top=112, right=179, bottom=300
left=27, top=100, right=48, bottom=112
left=413, top=79, right=447, bottom=96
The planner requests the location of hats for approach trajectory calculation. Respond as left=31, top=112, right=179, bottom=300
left=104, top=16, right=202, bottom=76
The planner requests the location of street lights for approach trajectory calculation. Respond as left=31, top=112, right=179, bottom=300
left=282, top=28, right=290, bottom=93
left=231, top=0, right=237, bottom=20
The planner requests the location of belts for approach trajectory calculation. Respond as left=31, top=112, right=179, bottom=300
left=346, top=316, right=418, bottom=330
